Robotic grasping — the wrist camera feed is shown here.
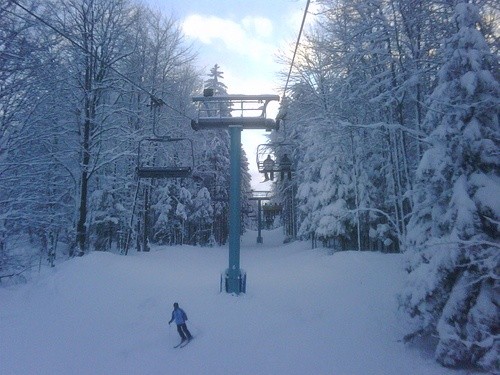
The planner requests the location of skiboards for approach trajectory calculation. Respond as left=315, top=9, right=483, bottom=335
left=174, top=335, right=193, bottom=348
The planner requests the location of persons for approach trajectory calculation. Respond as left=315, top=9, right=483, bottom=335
left=262, top=154, right=276, bottom=180
left=168, top=303, right=192, bottom=342
left=280, top=154, right=293, bottom=181
left=262, top=202, right=282, bottom=218
